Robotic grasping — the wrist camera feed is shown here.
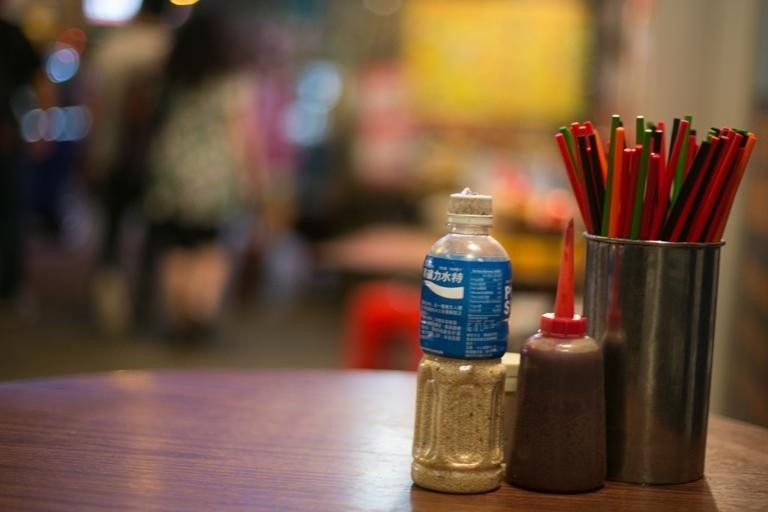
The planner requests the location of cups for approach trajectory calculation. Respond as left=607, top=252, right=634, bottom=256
left=582, top=234, right=724, bottom=485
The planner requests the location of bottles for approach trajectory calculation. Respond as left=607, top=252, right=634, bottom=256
left=514, top=215, right=606, bottom=495
left=413, top=193, right=513, bottom=493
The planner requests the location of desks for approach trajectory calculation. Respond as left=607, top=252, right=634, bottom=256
left=0, top=367, right=768, bottom=512
